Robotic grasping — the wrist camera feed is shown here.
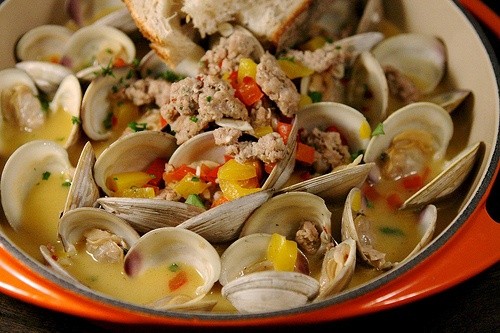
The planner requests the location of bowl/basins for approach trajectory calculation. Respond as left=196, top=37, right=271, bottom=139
left=0, top=0, right=500, bottom=322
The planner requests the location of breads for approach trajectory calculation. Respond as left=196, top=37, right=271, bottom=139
left=122, top=0, right=311, bottom=68
